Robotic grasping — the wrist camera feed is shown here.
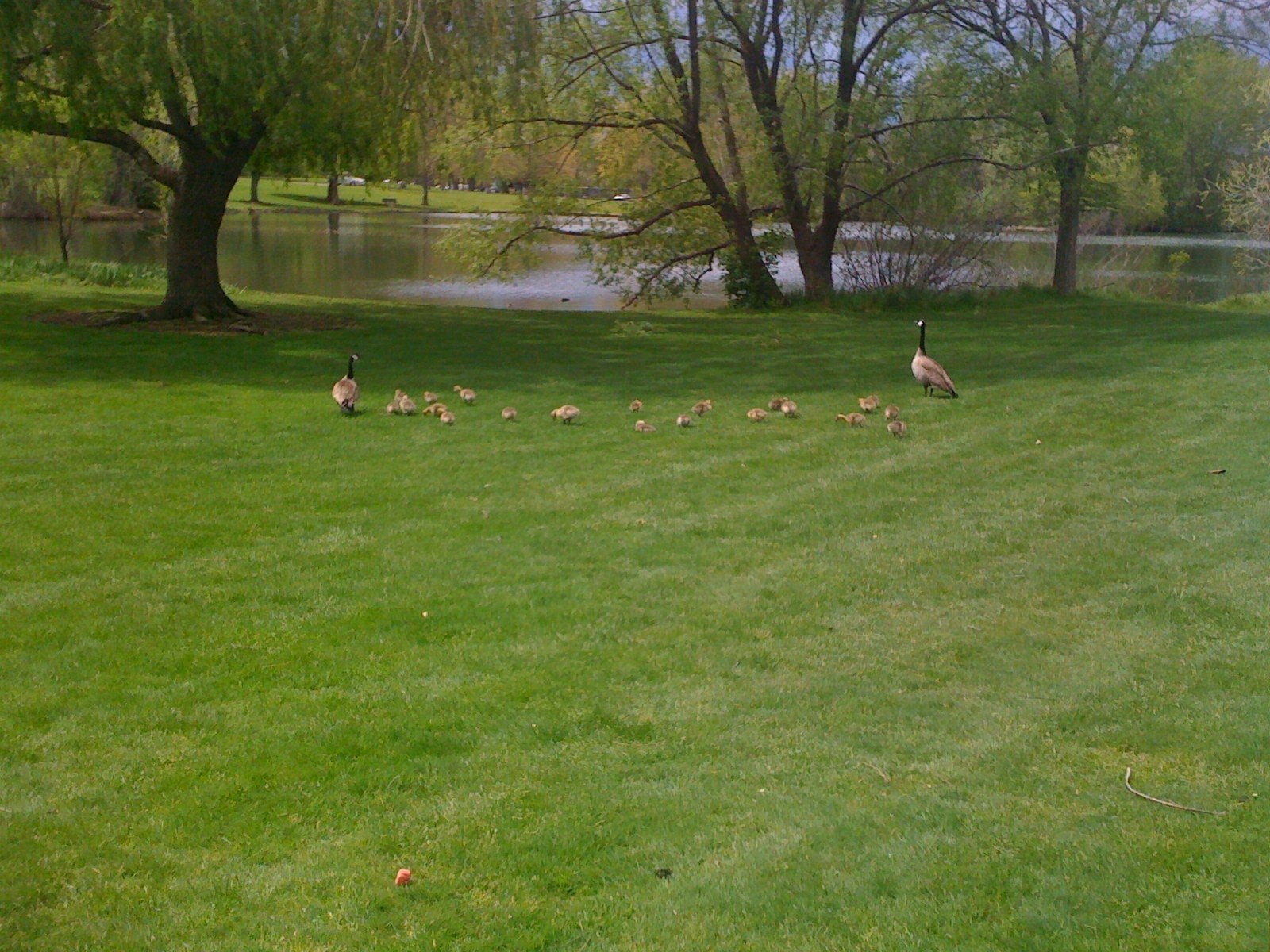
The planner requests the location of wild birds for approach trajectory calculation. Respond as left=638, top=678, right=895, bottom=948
left=550, top=405, right=580, bottom=424
left=635, top=421, right=655, bottom=432
left=745, top=408, right=767, bottom=423
left=691, top=399, right=713, bottom=418
left=887, top=421, right=907, bottom=436
left=910, top=319, right=959, bottom=399
left=677, top=414, right=689, bottom=428
left=884, top=403, right=899, bottom=420
left=835, top=413, right=865, bottom=428
left=331, top=353, right=363, bottom=413
left=501, top=406, right=516, bottom=422
left=386, top=390, right=415, bottom=417
left=769, top=397, right=796, bottom=418
left=629, top=399, right=642, bottom=412
left=436, top=408, right=454, bottom=426
left=423, top=391, right=438, bottom=404
left=423, top=403, right=447, bottom=416
left=453, top=386, right=475, bottom=405
left=560, top=298, right=569, bottom=302
left=858, top=395, right=879, bottom=413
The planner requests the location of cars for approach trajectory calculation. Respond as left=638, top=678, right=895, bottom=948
left=326, top=172, right=366, bottom=186
left=614, top=193, right=631, bottom=200
